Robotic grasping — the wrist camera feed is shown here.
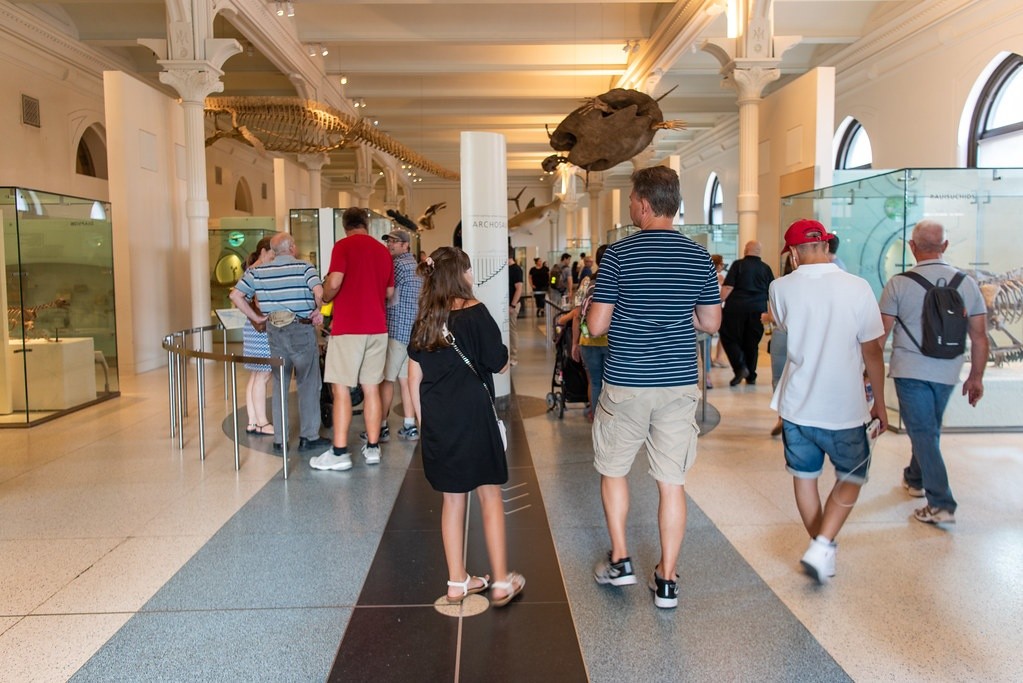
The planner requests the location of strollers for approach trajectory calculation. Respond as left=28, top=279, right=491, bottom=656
left=545, top=310, right=593, bottom=420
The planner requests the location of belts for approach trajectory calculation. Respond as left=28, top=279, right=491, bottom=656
left=267, top=317, right=312, bottom=324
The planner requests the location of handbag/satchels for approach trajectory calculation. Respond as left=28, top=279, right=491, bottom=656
left=269, top=311, right=297, bottom=328
left=246, top=301, right=265, bottom=332
left=496, top=418, right=509, bottom=451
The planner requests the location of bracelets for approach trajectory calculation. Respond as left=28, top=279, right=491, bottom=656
left=509, top=304, right=516, bottom=308
left=322, top=297, right=330, bottom=304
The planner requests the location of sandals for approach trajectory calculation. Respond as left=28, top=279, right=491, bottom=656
left=489, top=570, right=526, bottom=607
left=446, top=574, right=490, bottom=601
left=246, top=424, right=258, bottom=433
left=255, top=423, right=275, bottom=435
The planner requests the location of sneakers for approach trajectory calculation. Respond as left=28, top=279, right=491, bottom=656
left=360, top=443, right=381, bottom=464
left=902, top=477, right=926, bottom=497
left=594, top=550, right=638, bottom=586
left=914, top=504, right=956, bottom=524
left=799, top=537, right=840, bottom=581
left=647, top=563, right=680, bottom=609
left=398, top=425, right=420, bottom=441
left=309, top=450, right=352, bottom=470
left=360, top=427, right=390, bottom=442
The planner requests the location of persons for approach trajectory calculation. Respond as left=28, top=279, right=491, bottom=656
left=571, top=261, right=578, bottom=284
left=310, top=251, right=316, bottom=267
left=760, top=232, right=847, bottom=437
left=242, top=238, right=276, bottom=434
left=696, top=328, right=714, bottom=389
left=548, top=252, right=572, bottom=305
left=711, top=254, right=731, bottom=368
left=718, top=240, right=775, bottom=386
left=569, top=243, right=608, bottom=423
left=359, top=230, right=425, bottom=443
left=768, top=219, right=889, bottom=587
left=577, top=252, right=586, bottom=288
left=310, top=206, right=394, bottom=471
left=407, top=245, right=526, bottom=609
left=417, top=249, right=427, bottom=276
left=577, top=256, right=594, bottom=290
left=528, top=257, right=550, bottom=317
left=508, top=245, right=524, bottom=365
left=229, top=232, right=333, bottom=455
left=863, top=219, right=990, bottom=525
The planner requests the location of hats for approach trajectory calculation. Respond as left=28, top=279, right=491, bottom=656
left=781, top=219, right=834, bottom=254
left=382, top=229, right=411, bottom=242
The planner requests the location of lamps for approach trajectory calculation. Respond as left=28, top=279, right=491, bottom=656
left=726, top=0, right=745, bottom=38
left=247, top=42, right=253, bottom=57
left=352, top=98, right=366, bottom=108
left=274, top=0, right=295, bottom=18
left=622, top=39, right=641, bottom=53
left=17, top=189, right=29, bottom=211
left=308, top=42, right=328, bottom=57
left=339, top=72, right=347, bottom=84
left=90, top=201, right=105, bottom=220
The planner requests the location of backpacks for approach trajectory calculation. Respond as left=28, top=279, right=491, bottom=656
left=581, top=273, right=597, bottom=315
left=895, top=271, right=968, bottom=358
left=549, top=266, right=568, bottom=289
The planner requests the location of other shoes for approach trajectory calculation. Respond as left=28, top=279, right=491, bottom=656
left=747, top=378, right=755, bottom=384
left=299, top=436, right=331, bottom=451
left=730, top=367, right=749, bottom=386
left=536, top=309, right=545, bottom=317
left=707, top=381, right=713, bottom=389
left=272, top=442, right=289, bottom=454
left=510, top=360, right=517, bottom=365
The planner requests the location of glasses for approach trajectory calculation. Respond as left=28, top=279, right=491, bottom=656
left=386, top=239, right=405, bottom=243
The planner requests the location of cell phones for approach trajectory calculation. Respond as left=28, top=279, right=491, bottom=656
left=865, top=418, right=880, bottom=438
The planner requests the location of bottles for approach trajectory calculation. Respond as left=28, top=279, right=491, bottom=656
left=320, top=300, right=333, bottom=317
left=764, top=323, right=772, bottom=335
